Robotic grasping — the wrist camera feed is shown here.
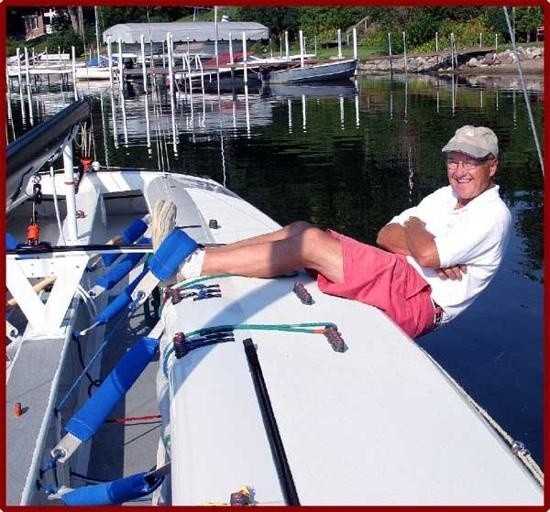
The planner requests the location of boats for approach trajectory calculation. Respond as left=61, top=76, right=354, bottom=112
left=2, top=101, right=544, bottom=506
left=5, top=49, right=359, bottom=96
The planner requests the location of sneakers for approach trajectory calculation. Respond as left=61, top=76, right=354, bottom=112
left=151, top=199, right=185, bottom=287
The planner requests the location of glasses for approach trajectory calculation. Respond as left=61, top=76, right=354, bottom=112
left=445, top=158, right=493, bottom=171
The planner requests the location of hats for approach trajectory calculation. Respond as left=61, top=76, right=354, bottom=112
left=441, top=125, right=499, bottom=159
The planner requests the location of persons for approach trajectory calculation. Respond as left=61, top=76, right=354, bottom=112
left=149, top=123, right=514, bottom=342
